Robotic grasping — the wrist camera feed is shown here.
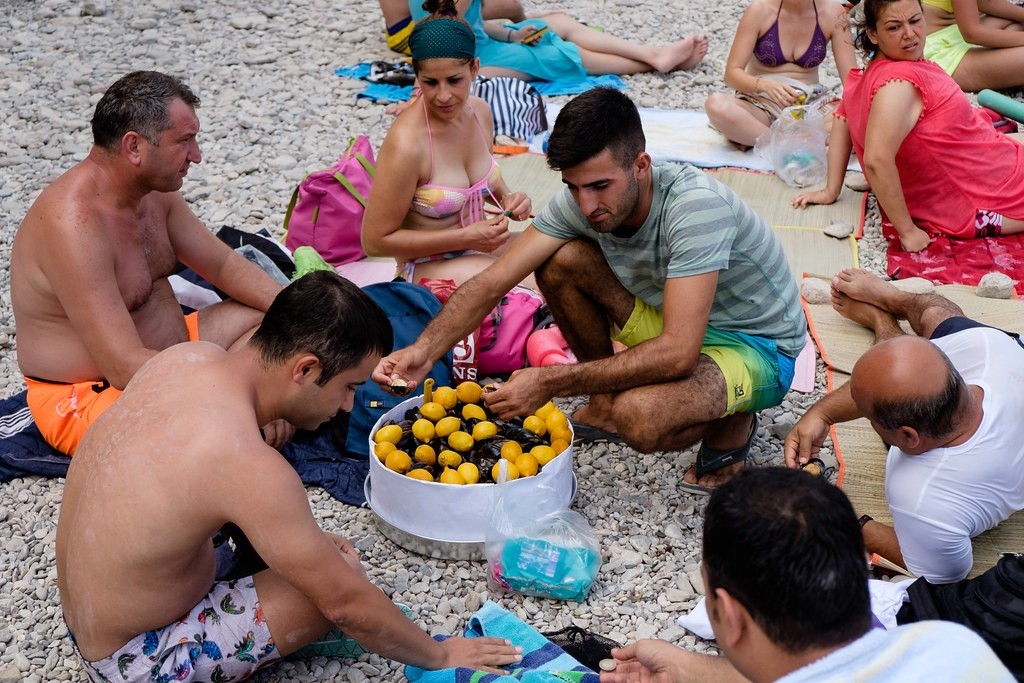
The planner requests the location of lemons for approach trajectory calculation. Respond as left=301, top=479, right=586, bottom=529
left=374, top=381, right=572, bottom=485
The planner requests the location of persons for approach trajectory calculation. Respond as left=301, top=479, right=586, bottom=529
left=918, top=0, right=1024, bottom=91
left=783, top=267, right=1023, bottom=589
left=599, top=465, right=1017, bottom=683
left=12, top=72, right=299, bottom=457
left=791, top=0, right=1024, bottom=254
left=56, top=268, right=523, bottom=683
left=377, top=1, right=527, bottom=59
left=370, top=89, right=808, bottom=496
left=705, top=0, right=863, bottom=155
left=377, top=0, right=709, bottom=83
left=359, top=21, right=543, bottom=304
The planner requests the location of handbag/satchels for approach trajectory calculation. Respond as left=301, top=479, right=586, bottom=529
left=477, top=285, right=548, bottom=382
left=418, top=278, right=481, bottom=386
left=469, top=75, right=548, bottom=141
left=896, top=551, right=1024, bottom=683
left=753, top=105, right=828, bottom=188
left=283, top=133, right=375, bottom=268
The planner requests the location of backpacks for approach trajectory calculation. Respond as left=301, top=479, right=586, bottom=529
left=335, top=276, right=454, bottom=458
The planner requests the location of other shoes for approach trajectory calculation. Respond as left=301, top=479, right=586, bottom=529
left=290, top=600, right=413, bottom=659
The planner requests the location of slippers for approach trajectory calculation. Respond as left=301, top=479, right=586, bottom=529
left=679, top=410, right=759, bottom=495
left=564, top=409, right=622, bottom=443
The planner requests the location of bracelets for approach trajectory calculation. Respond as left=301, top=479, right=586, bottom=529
left=506, top=29, right=515, bottom=44
left=857, top=514, right=874, bottom=527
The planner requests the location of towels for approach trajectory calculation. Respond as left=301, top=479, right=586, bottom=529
left=1, top=388, right=371, bottom=510
left=876, top=199, right=1024, bottom=295
left=527, top=103, right=770, bottom=172
left=404, top=598, right=600, bottom=683
